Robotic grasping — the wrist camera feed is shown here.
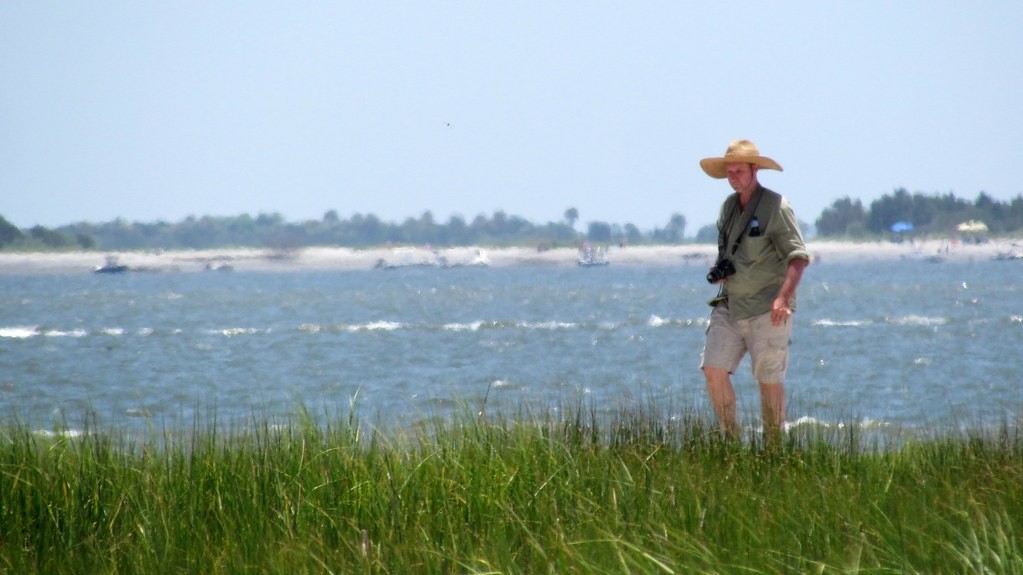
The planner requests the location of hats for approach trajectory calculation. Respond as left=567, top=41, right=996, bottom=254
left=699, top=140, right=783, bottom=179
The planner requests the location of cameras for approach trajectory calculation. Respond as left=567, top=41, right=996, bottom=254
left=706, top=258, right=736, bottom=284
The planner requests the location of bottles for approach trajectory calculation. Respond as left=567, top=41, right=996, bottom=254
left=749, top=215, right=761, bottom=237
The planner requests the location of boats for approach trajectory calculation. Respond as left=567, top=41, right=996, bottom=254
left=578, top=246, right=610, bottom=268
left=206, top=262, right=234, bottom=272
left=94, top=262, right=126, bottom=274
left=990, top=247, right=1023, bottom=261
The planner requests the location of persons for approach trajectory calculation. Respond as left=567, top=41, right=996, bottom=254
left=700, top=140, right=810, bottom=440
